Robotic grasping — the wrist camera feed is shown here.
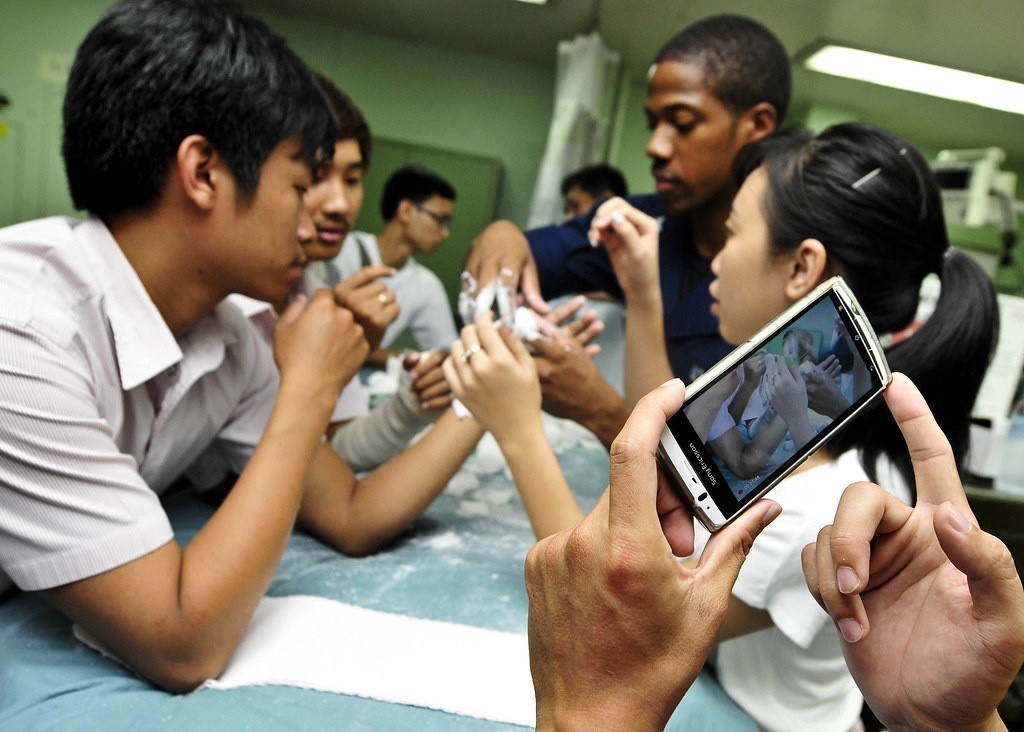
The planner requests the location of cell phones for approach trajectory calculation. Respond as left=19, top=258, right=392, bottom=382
left=658, top=277, right=894, bottom=533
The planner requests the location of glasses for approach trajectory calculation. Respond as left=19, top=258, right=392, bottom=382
left=416, top=205, right=452, bottom=224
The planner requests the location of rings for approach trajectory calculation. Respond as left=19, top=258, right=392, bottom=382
left=464, top=343, right=482, bottom=359
left=770, top=372, right=780, bottom=381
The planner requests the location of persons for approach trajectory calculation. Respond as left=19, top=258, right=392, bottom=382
left=524, top=370, right=1024, bottom=732
left=726, top=350, right=765, bottom=435
left=0, top=1, right=521, bottom=697
left=230, top=0, right=1024, bottom=731
left=687, top=347, right=841, bottom=482
left=765, top=362, right=850, bottom=451
